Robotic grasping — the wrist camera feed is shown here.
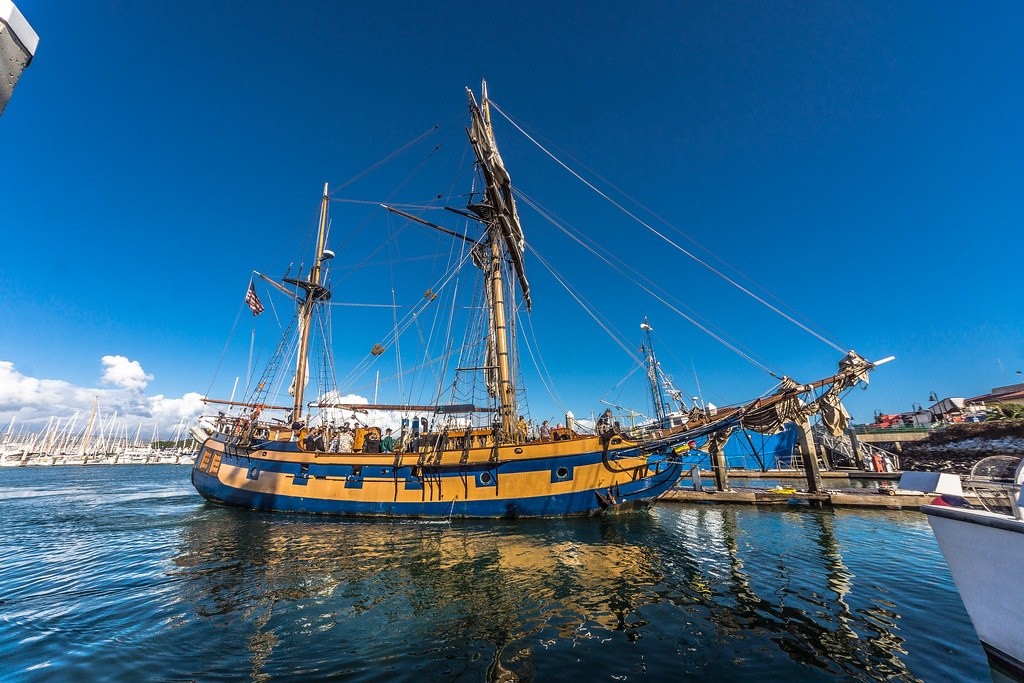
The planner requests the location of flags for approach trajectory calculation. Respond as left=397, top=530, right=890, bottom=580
left=246, top=278, right=265, bottom=318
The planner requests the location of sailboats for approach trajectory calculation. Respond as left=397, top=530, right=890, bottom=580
left=188, top=80, right=899, bottom=523
left=0, top=396, right=195, bottom=467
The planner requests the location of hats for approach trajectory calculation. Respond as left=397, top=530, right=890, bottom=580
left=386, top=428, right=392, bottom=431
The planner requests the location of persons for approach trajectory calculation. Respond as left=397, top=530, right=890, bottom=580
left=328, top=428, right=355, bottom=452
left=363, top=428, right=394, bottom=452
left=598, top=410, right=611, bottom=425
left=514, top=415, right=529, bottom=443
left=302, top=427, right=325, bottom=452
left=412, top=432, right=419, bottom=452
left=539, top=420, right=550, bottom=438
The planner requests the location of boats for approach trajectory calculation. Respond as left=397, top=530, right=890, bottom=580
left=918, top=453, right=1024, bottom=683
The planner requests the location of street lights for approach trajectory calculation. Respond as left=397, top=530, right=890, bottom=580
left=928, top=391, right=946, bottom=423
left=912, top=402, right=925, bottom=428
left=874, top=409, right=883, bottom=428
